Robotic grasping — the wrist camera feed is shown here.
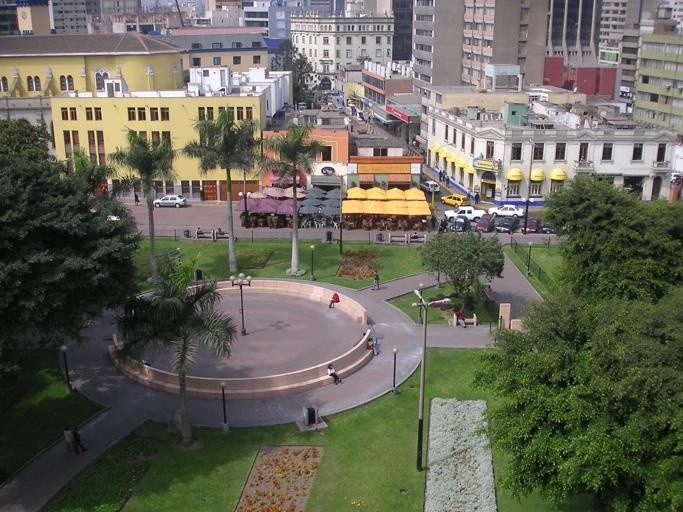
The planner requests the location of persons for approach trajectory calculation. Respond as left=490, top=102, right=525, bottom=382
left=475, top=192, right=479, bottom=205
left=443, top=170, right=446, bottom=182
left=446, top=176, right=450, bottom=188
left=73, top=426, right=88, bottom=455
left=457, top=310, right=465, bottom=328
left=64, top=425, right=75, bottom=453
left=439, top=170, right=443, bottom=181
left=135, top=193, right=139, bottom=206
left=328, top=364, right=341, bottom=385
left=368, top=335, right=378, bottom=356
left=328, top=292, right=339, bottom=308
left=431, top=215, right=438, bottom=229
left=371, top=270, right=379, bottom=291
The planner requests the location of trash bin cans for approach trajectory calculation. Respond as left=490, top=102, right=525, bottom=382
left=378, top=233, right=382, bottom=241
left=184, top=228, right=190, bottom=237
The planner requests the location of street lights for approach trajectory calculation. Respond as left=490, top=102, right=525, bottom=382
left=238, top=191, right=251, bottom=229
left=310, top=245, right=315, bottom=281
left=526, top=241, right=532, bottom=277
left=230, top=272, right=252, bottom=336
left=410, top=288, right=451, bottom=472
left=60, top=345, right=72, bottom=395
left=391, top=347, right=399, bottom=394
left=417, top=283, right=423, bottom=324
left=220, top=381, right=232, bottom=435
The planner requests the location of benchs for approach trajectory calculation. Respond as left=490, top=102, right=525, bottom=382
left=388, top=232, right=427, bottom=246
left=195, top=229, right=237, bottom=241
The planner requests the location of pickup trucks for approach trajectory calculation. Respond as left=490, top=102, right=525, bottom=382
left=444, top=206, right=486, bottom=222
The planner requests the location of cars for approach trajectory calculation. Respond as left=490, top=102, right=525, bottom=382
left=521, top=217, right=542, bottom=233
left=495, top=217, right=519, bottom=235
left=488, top=204, right=524, bottom=218
left=475, top=214, right=495, bottom=232
left=450, top=216, right=471, bottom=232
left=420, top=180, right=440, bottom=193
left=152, top=195, right=186, bottom=208
left=542, top=223, right=556, bottom=234
left=441, top=193, right=470, bottom=208
